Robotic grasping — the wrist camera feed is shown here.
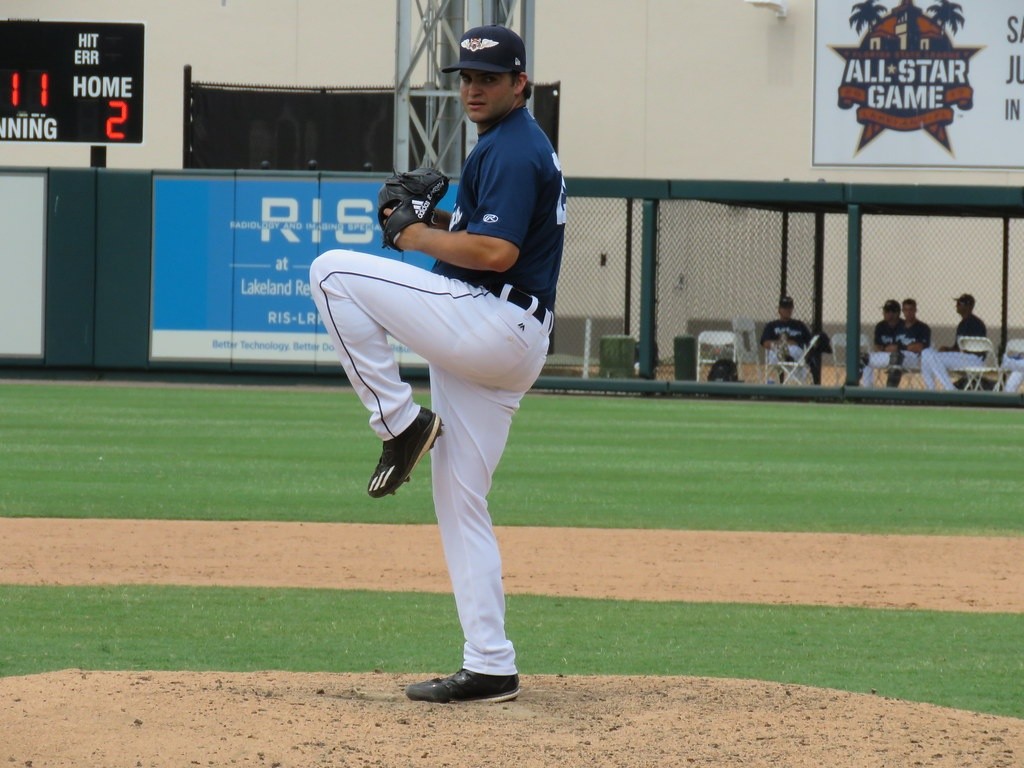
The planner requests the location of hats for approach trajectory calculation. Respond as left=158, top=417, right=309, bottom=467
left=780, top=297, right=794, bottom=308
left=881, top=300, right=900, bottom=312
left=953, top=294, right=975, bottom=307
left=442, top=25, right=527, bottom=75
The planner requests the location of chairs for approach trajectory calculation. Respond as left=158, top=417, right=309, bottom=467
left=697, top=331, right=819, bottom=386
left=831, top=332, right=1024, bottom=393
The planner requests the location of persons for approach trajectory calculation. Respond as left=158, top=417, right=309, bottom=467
left=309, top=26, right=567, bottom=705
left=921, top=294, right=986, bottom=391
left=860, top=299, right=931, bottom=368
left=862, top=300, right=904, bottom=389
left=761, top=297, right=811, bottom=384
left=1002, top=354, right=1024, bottom=393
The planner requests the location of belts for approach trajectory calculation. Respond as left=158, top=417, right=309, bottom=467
left=477, top=279, right=552, bottom=331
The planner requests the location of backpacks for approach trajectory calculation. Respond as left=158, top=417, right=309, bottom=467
left=707, top=358, right=738, bottom=399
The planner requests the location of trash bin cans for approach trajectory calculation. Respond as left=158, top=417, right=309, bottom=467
left=599, top=336, right=637, bottom=379
left=672, top=335, right=696, bottom=381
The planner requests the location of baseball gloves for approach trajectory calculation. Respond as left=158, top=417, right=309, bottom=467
left=377, top=168, right=450, bottom=253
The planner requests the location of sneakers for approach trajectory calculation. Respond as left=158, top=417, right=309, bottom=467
left=367, top=407, right=445, bottom=498
left=405, top=668, right=520, bottom=704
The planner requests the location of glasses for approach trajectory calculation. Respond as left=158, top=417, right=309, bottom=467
left=956, top=301, right=965, bottom=306
